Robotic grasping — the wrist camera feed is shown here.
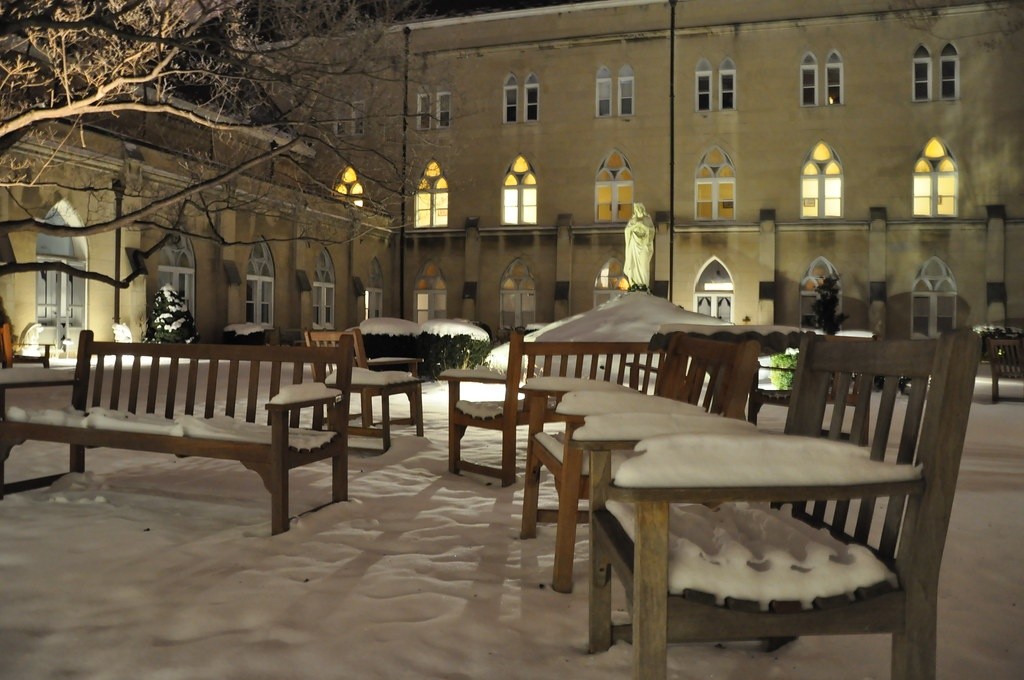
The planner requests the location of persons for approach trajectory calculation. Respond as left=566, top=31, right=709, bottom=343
left=622, top=200, right=656, bottom=293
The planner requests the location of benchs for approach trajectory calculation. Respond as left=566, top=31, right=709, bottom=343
left=0, top=329, right=353, bottom=534
left=440, top=330, right=691, bottom=489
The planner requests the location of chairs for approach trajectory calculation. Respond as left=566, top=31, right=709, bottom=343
left=986, top=336, right=1023, bottom=403
left=580, top=327, right=983, bottom=680
left=514, top=327, right=761, bottom=595
left=304, top=327, right=425, bottom=454
